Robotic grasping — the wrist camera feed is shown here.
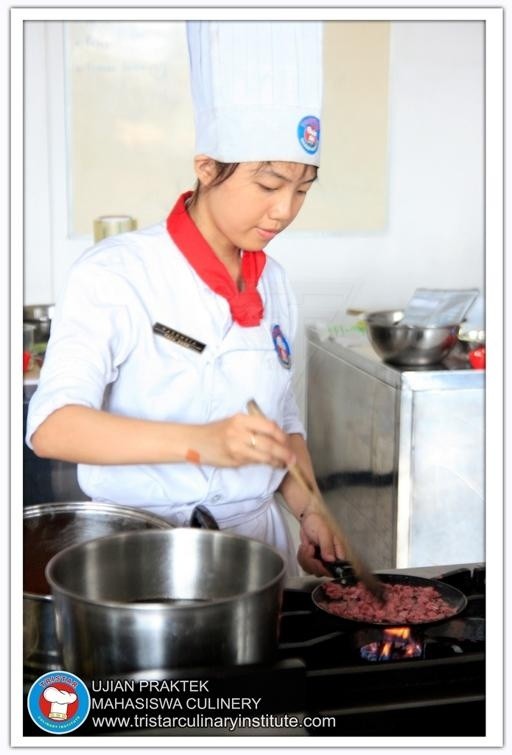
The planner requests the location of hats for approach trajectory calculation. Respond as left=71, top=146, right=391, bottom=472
left=188, top=20, right=321, bottom=168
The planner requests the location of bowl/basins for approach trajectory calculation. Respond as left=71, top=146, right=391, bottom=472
left=364, top=310, right=462, bottom=366
left=23, top=303, right=53, bottom=346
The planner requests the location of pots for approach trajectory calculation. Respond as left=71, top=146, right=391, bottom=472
left=22, top=501, right=222, bottom=683
left=310, top=544, right=466, bottom=630
left=46, top=529, right=287, bottom=719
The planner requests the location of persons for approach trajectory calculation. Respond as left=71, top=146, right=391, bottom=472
left=24, top=20, right=345, bottom=591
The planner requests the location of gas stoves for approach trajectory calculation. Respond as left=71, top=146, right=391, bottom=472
left=279, top=561, right=487, bottom=724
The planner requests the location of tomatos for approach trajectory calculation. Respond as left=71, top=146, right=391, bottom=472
left=468, top=347, right=485, bottom=369
